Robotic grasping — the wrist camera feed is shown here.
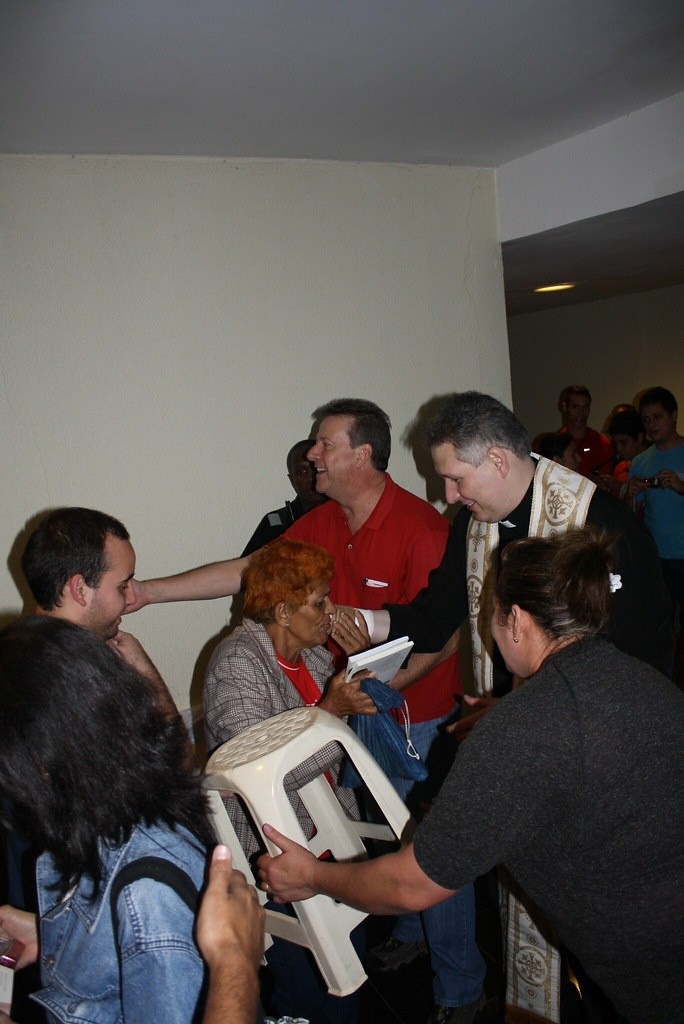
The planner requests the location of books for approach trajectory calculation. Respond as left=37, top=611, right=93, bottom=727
left=344, top=636, right=414, bottom=686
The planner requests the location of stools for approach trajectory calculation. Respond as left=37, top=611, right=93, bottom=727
left=204, top=706, right=411, bottom=999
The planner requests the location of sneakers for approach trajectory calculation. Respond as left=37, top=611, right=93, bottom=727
left=366, top=938, right=430, bottom=974
left=427, top=989, right=487, bottom=1024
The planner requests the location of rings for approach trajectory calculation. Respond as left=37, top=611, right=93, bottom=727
left=266, top=885, right=270, bottom=893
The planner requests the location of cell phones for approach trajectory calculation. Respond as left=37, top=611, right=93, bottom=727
left=0, top=927, right=25, bottom=969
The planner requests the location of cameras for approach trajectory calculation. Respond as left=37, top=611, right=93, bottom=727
left=641, top=477, right=662, bottom=489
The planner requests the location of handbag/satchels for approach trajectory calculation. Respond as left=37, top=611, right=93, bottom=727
left=341, top=678, right=429, bottom=789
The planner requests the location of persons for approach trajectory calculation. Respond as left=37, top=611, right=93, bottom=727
left=256, top=531, right=684, bottom=1024
left=535, top=386, right=684, bottom=690
left=327, top=391, right=675, bottom=1024
left=244, top=440, right=332, bottom=557
left=125, top=397, right=490, bottom=1024
left=204, top=544, right=379, bottom=1023
left=0, top=507, right=266, bottom=1024
